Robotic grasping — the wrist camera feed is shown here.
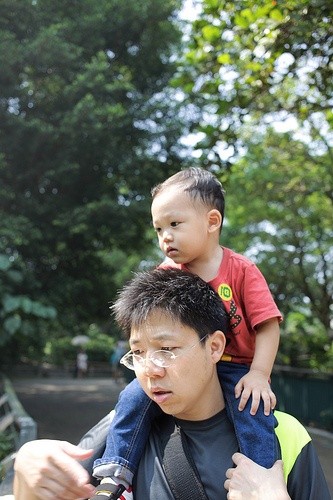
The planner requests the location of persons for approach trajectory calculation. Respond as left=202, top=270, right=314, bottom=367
left=12, top=265, right=331, bottom=500
left=88, top=166, right=284, bottom=500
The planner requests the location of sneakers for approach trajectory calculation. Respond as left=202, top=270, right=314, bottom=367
left=90, top=483, right=133, bottom=500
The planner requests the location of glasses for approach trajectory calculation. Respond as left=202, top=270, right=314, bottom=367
left=120, top=334, right=209, bottom=370
